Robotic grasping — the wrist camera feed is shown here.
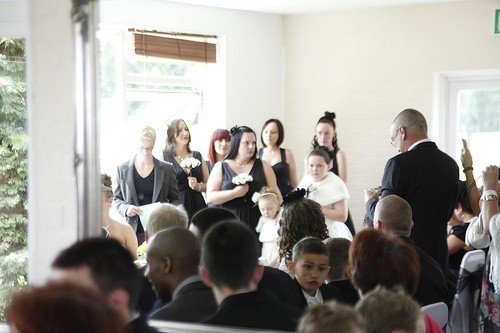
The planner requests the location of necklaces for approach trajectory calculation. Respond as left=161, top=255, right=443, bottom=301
left=175, top=153, right=190, bottom=160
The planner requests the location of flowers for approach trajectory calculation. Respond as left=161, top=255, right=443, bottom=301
left=179, top=157, right=203, bottom=177
left=232, top=173, right=254, bottom=204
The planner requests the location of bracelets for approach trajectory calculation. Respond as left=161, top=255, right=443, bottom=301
left=463, top=167, right=473, bottom=173
left=197, top=183, right=203, bottom=193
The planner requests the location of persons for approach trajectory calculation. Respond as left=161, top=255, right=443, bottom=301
left=459, top=140, right=482, bottom=216
left=5, top=186, right=500, bottom=333
left=296, top=146, right=353, bottom=242
left=205, top=129, right=232, bottom=174
left=205, top=126, right=283, bottom=228
left=100, top=172, right=139, bottom=261
left=257, top=120, right=299, bottom=196
left=158, top=119, right=210, bottom=225
left=478, top=164, right=500, bottom=333
left=446, top=181, right=475, bottom=271
left=363, top=110, right=460, bottom=269
left=113, top=127, right=184, bottom=246
left=311, top=111, right=356, bottom=238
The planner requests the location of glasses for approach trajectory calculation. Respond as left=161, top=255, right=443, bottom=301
left=391, top=126, right=401, bottom=146
